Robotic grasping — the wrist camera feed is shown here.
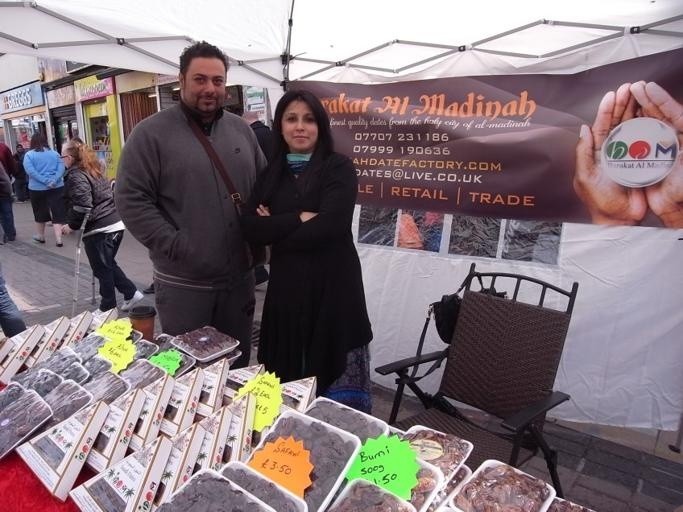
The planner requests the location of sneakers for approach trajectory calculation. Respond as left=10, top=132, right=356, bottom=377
left=121, top=290, right=145, bottom=311
left=90, top=307, right=119, bottom=316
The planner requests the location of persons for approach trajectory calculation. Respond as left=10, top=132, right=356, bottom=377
left=0, top=264, right=26, bottom=339
left=571, top=79, right=682, bottom=230
left=112, top=41, right=269, bottom=372
left=0, top=132, right=83, bottom=248
left=241, top=109, right=275, bottom=166
left=56, top=138, right=144, bottom=318
left=241, top=91, right=377, bottom=415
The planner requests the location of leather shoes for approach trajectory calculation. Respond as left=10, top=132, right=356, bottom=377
left=33, top=234, right=45, bottom=243
left=143, top=284, right=155, bottom=294
left=56, top=238, right=62, bottom=247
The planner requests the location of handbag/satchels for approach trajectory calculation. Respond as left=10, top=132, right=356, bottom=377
left=238, top=205, right=270, bottom=285
left=431, top=273, right=508, bottom=345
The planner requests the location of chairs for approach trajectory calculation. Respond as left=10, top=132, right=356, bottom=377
left=373, top=266, right=580, bottom=502
